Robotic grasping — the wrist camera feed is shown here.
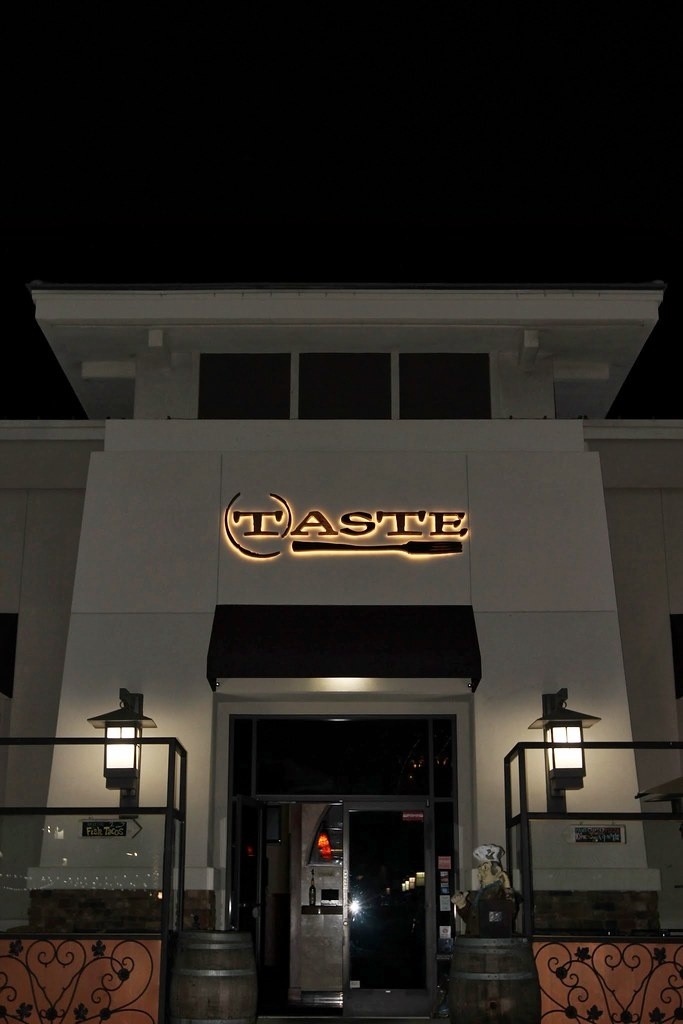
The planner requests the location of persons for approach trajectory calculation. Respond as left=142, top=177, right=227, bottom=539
left=450, top=845, right=520, bottom=933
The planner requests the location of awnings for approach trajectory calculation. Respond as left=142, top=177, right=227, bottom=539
left=205, top=605, right=483, bottom=693
left=0, top=612, right=19, bottom=699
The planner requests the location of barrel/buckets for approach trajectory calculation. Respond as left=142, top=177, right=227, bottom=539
left=448, top=935, right=542, bottom=1024
left=170, top=930, right=257, bottom=1024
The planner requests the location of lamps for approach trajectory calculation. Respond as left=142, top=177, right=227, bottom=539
left=525, top=685, right=604, bottom=820
left=86, top=686, right=161, bottom=823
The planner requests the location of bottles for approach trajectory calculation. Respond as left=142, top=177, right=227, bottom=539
left=309, top=879, right=316, bottom=906
